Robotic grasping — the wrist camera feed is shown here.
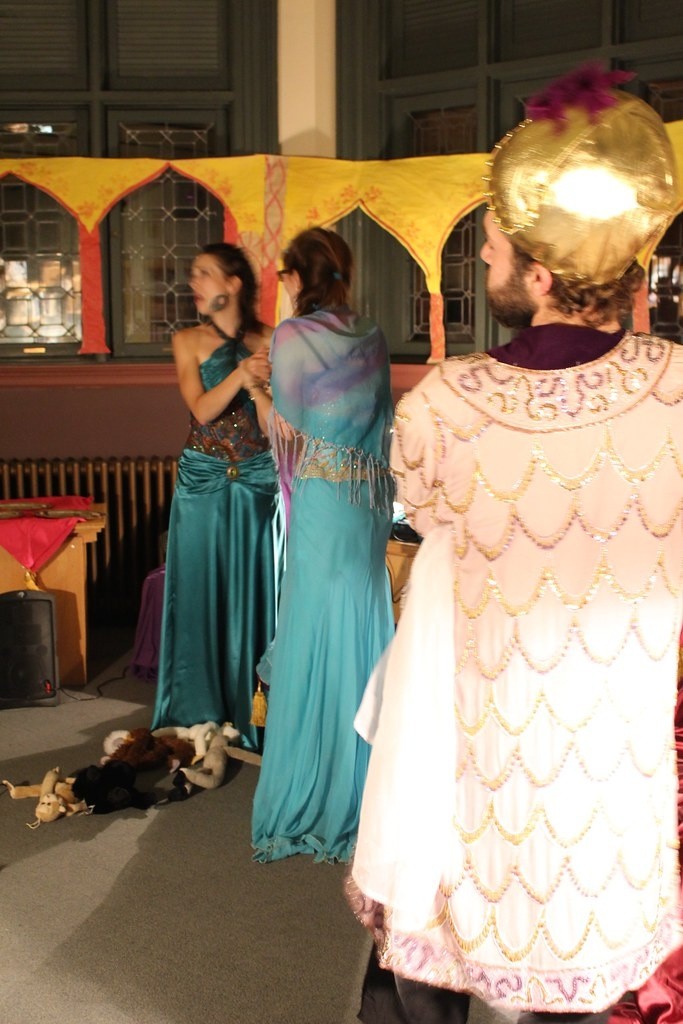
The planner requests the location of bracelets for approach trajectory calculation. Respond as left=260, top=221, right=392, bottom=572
left=246, top=384, right=262, bottom=389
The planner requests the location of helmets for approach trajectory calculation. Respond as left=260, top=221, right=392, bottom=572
left=488, top=72, right=683, bottom=282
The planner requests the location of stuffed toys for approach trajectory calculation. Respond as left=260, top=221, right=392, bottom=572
left=2, top=721, right=239, bottom=830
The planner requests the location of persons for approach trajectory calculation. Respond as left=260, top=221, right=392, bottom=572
left=151, top=242, right=290, bottom=756
left=344, top=62, right=683, bottom=1024
left=239, top=226, right=393, bottom=861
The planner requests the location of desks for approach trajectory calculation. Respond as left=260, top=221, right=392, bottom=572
left=0, top=500, right=107, bottom=685
